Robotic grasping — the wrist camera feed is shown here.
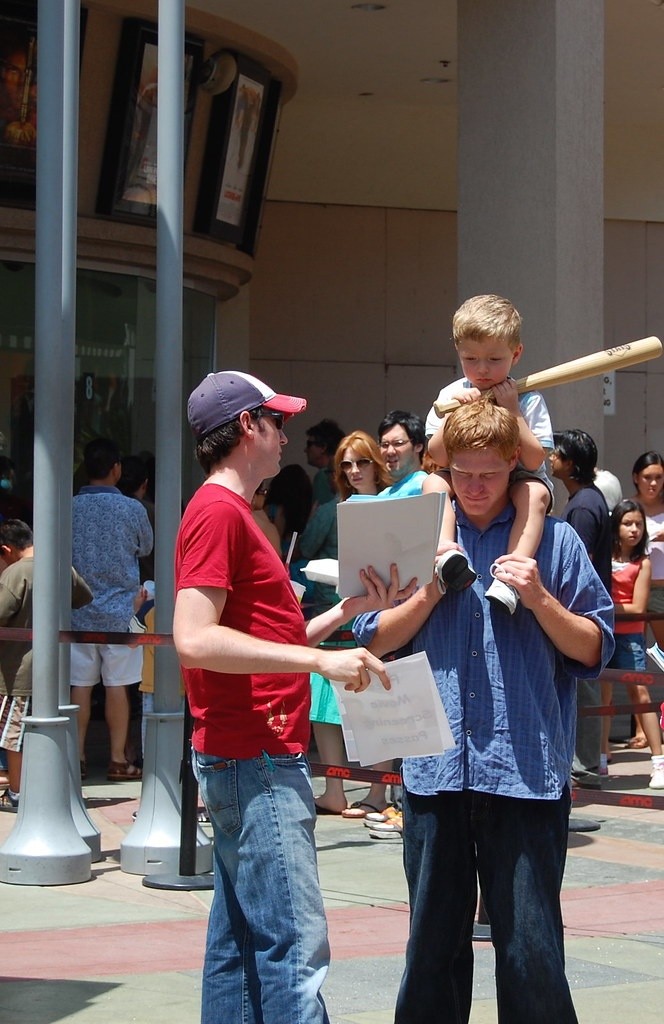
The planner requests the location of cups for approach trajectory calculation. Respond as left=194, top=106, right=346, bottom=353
left=142, top=580, right=156, bottom=601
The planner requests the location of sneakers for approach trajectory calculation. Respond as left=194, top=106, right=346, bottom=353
left=0, top=789, right=19, bottom=812
left=648, top=761, right=664, bottom=788
left=434, top=549, right=477, bottom=595
left=599, top=767, right=609, bottom=775
left=369, top=811, right=403, bottom=839
left=362, top=797, right=403, bottom=827
left=484, top=563, right=520, bottom=615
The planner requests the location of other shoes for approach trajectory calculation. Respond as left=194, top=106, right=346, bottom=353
left=628, top=737, right=649, bottom=749
left=0, top=775, right=11, bottom=790
left=570, top=778, right=601, bottom=790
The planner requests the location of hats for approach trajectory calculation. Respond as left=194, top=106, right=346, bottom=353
left=187, top=370, right=308, bottom=439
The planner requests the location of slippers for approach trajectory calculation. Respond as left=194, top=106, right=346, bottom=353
left=314, top=797, right=341, bottom=815
left=343, top=801, right=382, bottom=818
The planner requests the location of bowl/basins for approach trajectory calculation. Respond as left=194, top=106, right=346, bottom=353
left=300, top=559, right=339, bottom=586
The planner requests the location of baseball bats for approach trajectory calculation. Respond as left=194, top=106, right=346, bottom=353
left=433, top=335, right=664, bottom=419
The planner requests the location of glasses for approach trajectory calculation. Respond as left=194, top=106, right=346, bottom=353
left=380, top=438, right=413, bottom=449
left=260, top=411, right=285, bottom=430
left=340, top=458, right=375, bottom=471
left=258, top=489, right=268, bottom=497
left=307, top=440, right=319, bottom=448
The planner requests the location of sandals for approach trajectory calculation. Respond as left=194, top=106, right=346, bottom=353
left=107, top=759, right=142, bottom=781
left=80, top=760, right=87, bottom=779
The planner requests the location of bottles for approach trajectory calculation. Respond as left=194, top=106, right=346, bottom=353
left=289, top=580, right=306, bottom=606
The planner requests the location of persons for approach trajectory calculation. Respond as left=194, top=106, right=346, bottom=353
left=173, top=370, right=417, bottom=1024
left=235, top=85, right=260, bottom=169
left=121, top=450, right=193, bottom=780
left=0, top=519, right=94, bottom=811
left=421, top=294, right=550, bottom=614
left=351, top=400, right=613, bottom=1024
left=71, top=440, right=153, bottom=780
left=253, top=409, right=441, bottom=839
left=625, top=452, right=664, bottom=748
left=600, top=500, right=664, bottom=788
left=550, top=426, right=622, bottom=788
left=0, top=456, right=33, bottom=788
